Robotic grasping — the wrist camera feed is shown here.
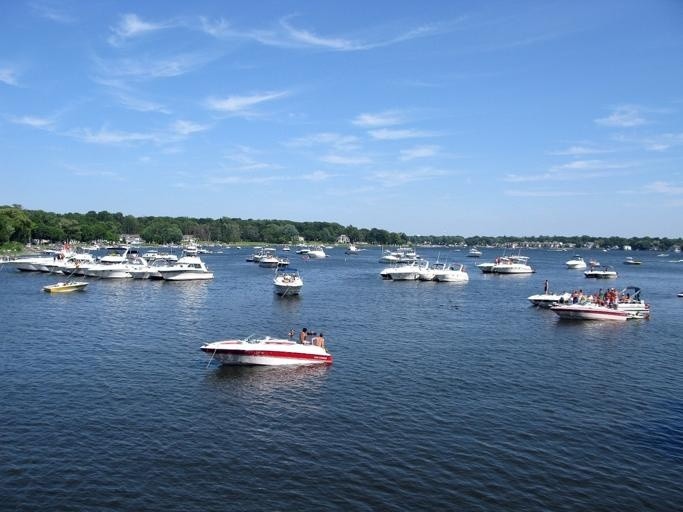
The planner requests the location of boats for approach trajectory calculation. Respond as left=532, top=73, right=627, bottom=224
left=199, top=337, right=333, bottom=365
left=379, top=246, right=469, bottom=282
left=590, top=260, right=600, bottom=266
left=43, top=282, right=88, bottom=293
left=274, top=266, right=303, bottom=296
left=476, top=255, right=534, bottom=273
left=566, top=257, right=587, bottom=269
left=253, top=246, right=291, bottom=268
left=526, top=285, right=650, bottom=321
left=296, top=244, right=326, bottom=259
left=468, top=249, right=482, bottom=257
left=11, top=237, right=214, bottom=281
left=624, top=260, right=642, bottom=265
left=347, top=243, right=361, bottom=252
left=583, top=271, right=617, bottom=279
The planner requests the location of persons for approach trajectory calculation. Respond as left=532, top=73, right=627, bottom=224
left=571, top=287, right=630, bottom=308
left=543, top=279, right=548, bottom=295
left=495, top=256, right=498, bottom=266
left=314, top=332, right=324, bottom=349
left=52, top=252, right=56, bottom=262
left=60, top=252, right=64, bottom=260
left=298, top=327, right=307, bottom=345
left=558, top=296, right=564, bottom=304
left=57, top=254, right=59, bottom=259
